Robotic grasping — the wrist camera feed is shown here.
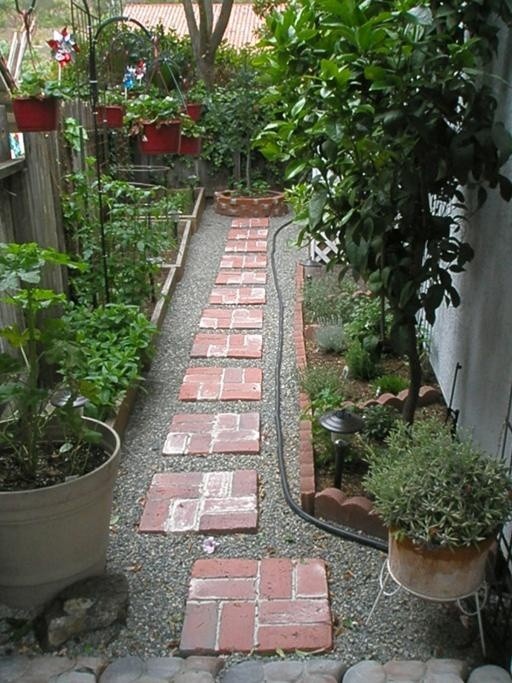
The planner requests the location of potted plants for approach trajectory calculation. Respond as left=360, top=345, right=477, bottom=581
left=0, top=240, right=163, bottom=626
left=354, top=423, right=510, bottom=602
left=8, top=46, right=74, bottom=130
left=93, top=85, right=208, bottom=156
